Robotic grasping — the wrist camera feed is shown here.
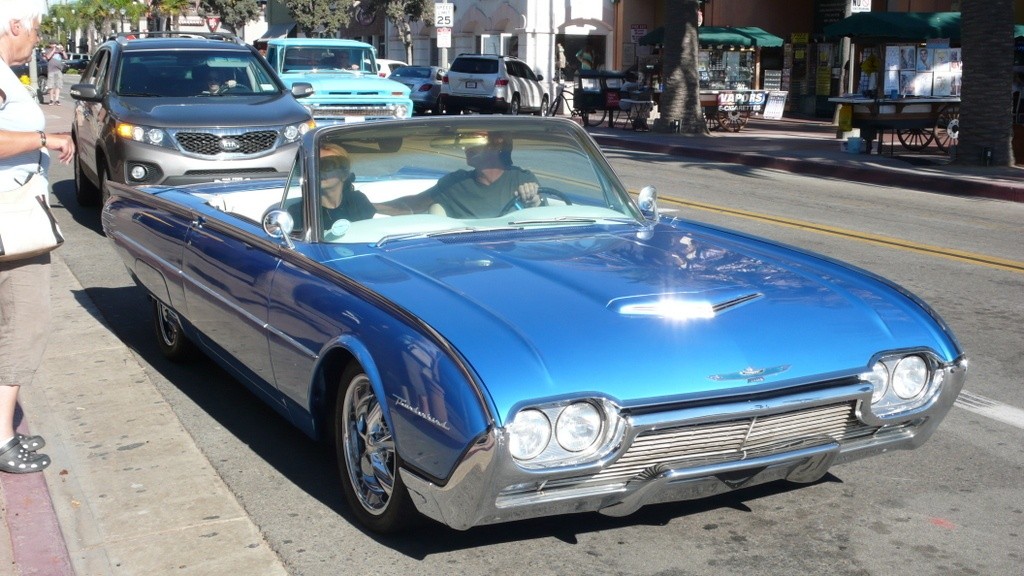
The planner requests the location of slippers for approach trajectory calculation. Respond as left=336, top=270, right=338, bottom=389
left=15, top=434, right=46, bottom=452
left=0, top=438, right=50, bottom=474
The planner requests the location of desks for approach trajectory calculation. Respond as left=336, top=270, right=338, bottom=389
left=620, top=99, right=656, bottom=131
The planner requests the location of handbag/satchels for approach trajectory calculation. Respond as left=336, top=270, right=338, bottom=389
left=0, top=150, right=65, bottom=261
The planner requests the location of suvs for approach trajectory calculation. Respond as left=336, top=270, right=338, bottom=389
left=71, top=32, right=318, bottom=219
left=364, top=59, right=408, bottom=79
left=441, top=53, right=551, bottom=116
left=253, top=38, right=414, bottom=153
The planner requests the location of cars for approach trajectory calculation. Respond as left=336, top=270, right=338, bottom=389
left=10, top=53, right=69, bottom=95
left=388, top=66, right=448, bottom=114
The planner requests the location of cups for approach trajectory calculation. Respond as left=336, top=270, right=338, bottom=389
left=891, top=90, right=897, bottom=99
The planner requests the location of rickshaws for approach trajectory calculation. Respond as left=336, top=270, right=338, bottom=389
left=636, top=25, right=785, bottom=133
left=826, top=10, right=1024, bottom=156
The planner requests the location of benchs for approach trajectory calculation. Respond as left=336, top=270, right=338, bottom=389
left=195, top=178, right=449, bottom=224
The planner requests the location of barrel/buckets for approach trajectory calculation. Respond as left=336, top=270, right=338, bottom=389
left=848, top=137, right=861, bottom=155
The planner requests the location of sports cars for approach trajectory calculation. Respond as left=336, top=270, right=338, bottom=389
left=101, top=115, right=969, bottom=537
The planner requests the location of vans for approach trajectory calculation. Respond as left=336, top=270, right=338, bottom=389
left=67, top=53, right=93, bottom=61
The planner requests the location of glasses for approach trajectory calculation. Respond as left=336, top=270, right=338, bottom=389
left=319, top=158, right=343, bottom=171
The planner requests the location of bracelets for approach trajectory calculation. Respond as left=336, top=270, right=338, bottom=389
left=538, top=196, right=545, bottom=207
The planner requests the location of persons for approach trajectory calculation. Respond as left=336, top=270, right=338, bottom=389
left=919, top=49, right=930, bottom=70
left=201, top=69, right=237, bottom=95
left=35, top=48, right=47, bottom=104
left=843, top=59, right=861, bottom=94
left=1, top=0, right=75, bottom=474
left=288, top=143, right=377, bottom=238
left=372, top=132, right=548, bottom=219
left=332, top=52, right=360, bottom=70
left=45, top=40, right=68, bottom=105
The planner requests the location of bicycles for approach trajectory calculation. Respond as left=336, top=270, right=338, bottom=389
left=545, top=79, right=607, bottom=127
